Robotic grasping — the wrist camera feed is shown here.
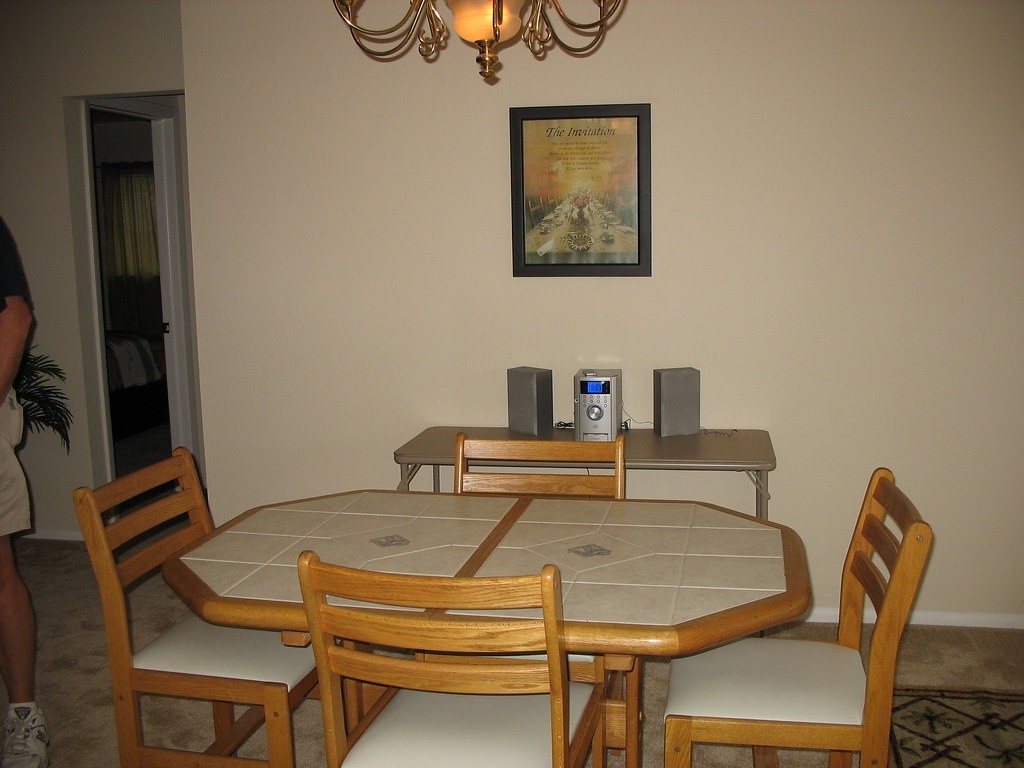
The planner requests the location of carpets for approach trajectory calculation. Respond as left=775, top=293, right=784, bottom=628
left=889, top=685, right=1024, bottom=768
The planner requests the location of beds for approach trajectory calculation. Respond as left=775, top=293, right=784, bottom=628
left=105, top=330, right=166, bottom=442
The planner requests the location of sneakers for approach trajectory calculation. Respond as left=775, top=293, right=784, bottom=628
left=0, top=705, right=50, bottom=768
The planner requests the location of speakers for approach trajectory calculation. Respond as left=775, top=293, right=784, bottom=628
left=654, top=367, right=700, bottom=436
left=507, top=366, right=553, bottom=435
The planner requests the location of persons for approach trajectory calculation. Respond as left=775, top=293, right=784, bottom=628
left=0, top=214, right=52, bottom=768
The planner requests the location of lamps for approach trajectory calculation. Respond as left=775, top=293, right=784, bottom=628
left=332, top=0, right=623, bottom=81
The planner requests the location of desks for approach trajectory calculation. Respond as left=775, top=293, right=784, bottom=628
left=393, top=426, right=776, bottom=519
left=164, top=489, right=817, bottom=768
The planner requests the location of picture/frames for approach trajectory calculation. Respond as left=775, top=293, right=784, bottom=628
left=509, top=101, right=652, bottom=277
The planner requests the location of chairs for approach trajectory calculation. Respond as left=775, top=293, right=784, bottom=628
left=453, top=433, right=640, bottom=756
left=73, top=447, right=375, bottom=768
left=663, top=468, right=935, bottom=768
left=298, top=546, right=613, bottom=767
